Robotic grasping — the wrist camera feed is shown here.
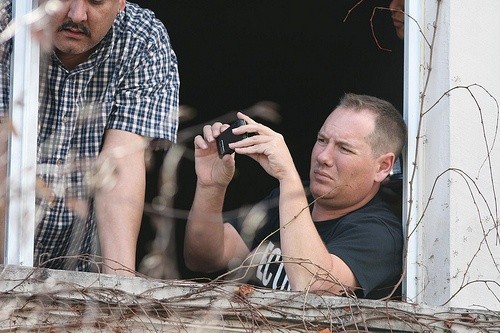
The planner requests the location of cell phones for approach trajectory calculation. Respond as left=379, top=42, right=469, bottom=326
left=216, top=119, right=248, bottom=158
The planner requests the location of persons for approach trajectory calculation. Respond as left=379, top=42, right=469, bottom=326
left=185, top=93, right=403, bottom=306
left=0, top=0, right=181, bottom=278
